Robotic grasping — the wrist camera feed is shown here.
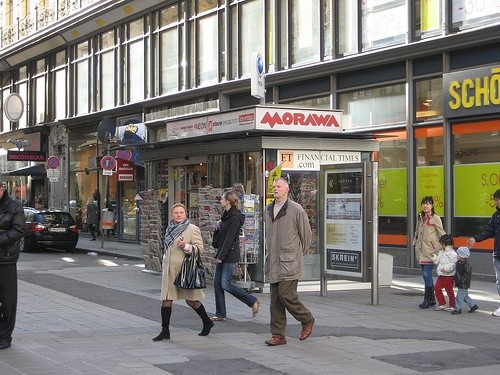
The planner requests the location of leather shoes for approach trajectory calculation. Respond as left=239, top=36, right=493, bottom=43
left=265, top=336, right=287, bottom=345
left=299, top=318, right=315, bottom=340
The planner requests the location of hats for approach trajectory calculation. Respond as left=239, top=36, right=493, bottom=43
left=456, top=245, right=470, bottom=258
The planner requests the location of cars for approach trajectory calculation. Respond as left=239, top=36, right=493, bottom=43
left=19, top=212, right=79, bottom=253
left=23, top=207, right=40, bottom=213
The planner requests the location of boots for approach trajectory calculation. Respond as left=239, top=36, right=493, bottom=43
left=428, top=285, right=437, bottom=305
left=195, top=303, right=214, bottom=336
left=152, top=306, right=172, bottom=341
left=419, top=286, right=432, bottom=308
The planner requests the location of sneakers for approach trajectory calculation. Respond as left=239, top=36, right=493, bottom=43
left=492, top=306, right=500, bottom=316
left=445, top=307, right=455, bottom=312
left=435, top=305, right=447, bottom=310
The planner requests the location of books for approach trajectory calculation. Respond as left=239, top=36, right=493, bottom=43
left=239, top=194, right=260, bottom=262
left=199, top=188, right=224, bottom=280
left=190, top=192, right=199, bottom=208
left=297, top=176, right=317, bottom=254
left=189, top=209, right=199, bottom=226
left=237, top=265, right=251, bottom=281
left=139, top=190, right=163, bottom=271
left=287, top=175, right=295, bottom=201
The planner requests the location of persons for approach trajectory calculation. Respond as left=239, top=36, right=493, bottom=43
left=210, top=191, right=261, bottom=321
left=15, top=189, right=21, bottom=201
left=451, top=246, right=478, bottom=314
left=432, top=234, right=459, bottom=311
left=153, top=203, right=214, bottom=341
left=413, top=196, right=447, bottom=309
left=262, top=178, right=315, bottom=345
left=86, top=197, right=101, bottom=241
left=465, top=189, right=500, bottom=316
left=191, top=173, right=199, bottom=186
left=0, top=182, right=25, bottom=350
left=201, top=175, right=208, bottom=188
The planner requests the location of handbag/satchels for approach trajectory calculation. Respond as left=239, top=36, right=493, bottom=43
left=212, top=225, right=220, bottom=248
left=174, top=245, right=206, bottom=289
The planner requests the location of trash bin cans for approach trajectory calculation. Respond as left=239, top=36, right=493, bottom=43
left=99, top=208, right=115, bottom=229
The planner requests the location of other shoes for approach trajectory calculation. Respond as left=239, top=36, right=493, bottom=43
left=0, top=342, right=11, bottom=350
left=468, top=304, right=478, bottom=313
left=210, top=316, right=226, bottom=321
left=89, top=239, right=96, bottom=241
left=96, top=231, right=101, bottom=237
left=451, top=309, right=461, bottom=315
left=253, top=298, right=260, bottom=317
